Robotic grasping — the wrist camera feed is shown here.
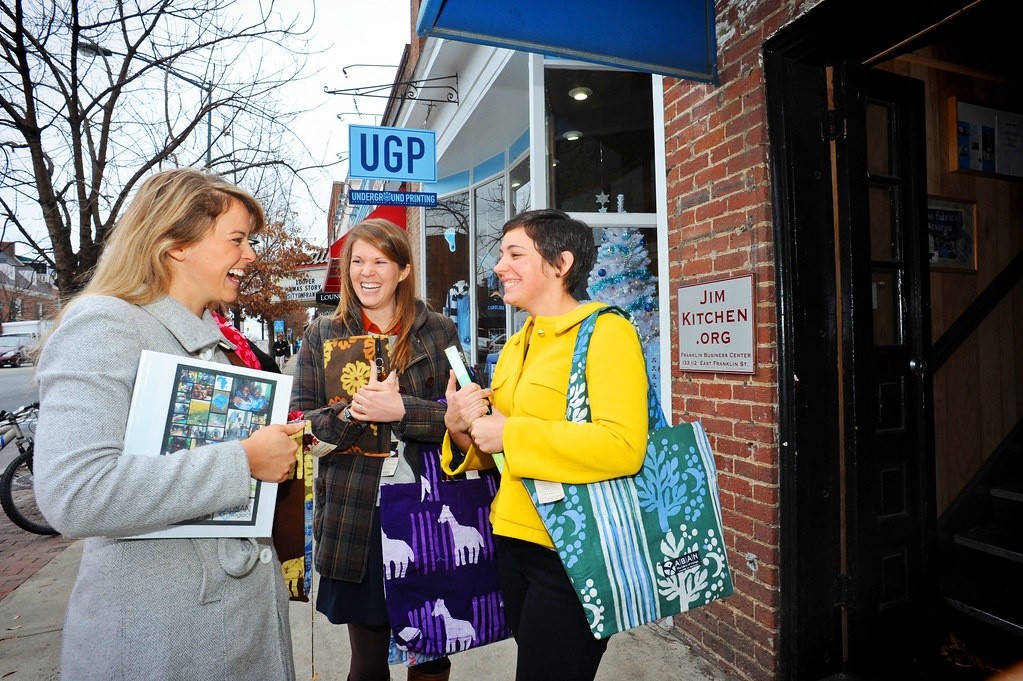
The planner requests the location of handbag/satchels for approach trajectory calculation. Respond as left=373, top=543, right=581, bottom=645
left=380, top=397, right=511, bottom=655
left=221, top=344, right=315, bottom=603
left=519, top=305, right=734, bottom=640
left=443, top=291, right=450, bottom=318
left=323, top=333, right=390, bottom=457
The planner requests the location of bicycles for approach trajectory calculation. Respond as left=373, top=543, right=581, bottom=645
left=0, top=398, right=61, bottom=537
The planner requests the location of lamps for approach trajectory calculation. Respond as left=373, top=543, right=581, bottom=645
left=568, top=85, right=592, bottom=100
left=563, top=131, right=583, bottom=140
left=553, top=160, right=560, bottom=167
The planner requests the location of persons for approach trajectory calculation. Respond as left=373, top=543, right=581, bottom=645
left=285, top=219, right=483, bottom=681
left=445, top=279, right=481, bottom=368
left=34, top=166, right=307, bottom=681
left=164, top=368, right=269, bottom=454
left=272, top=335, right=288, bottom=374
left=440, top=208, right=650, bottom=681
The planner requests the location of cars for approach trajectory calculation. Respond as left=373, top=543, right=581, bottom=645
left=0, top=332, right=40, bottom=368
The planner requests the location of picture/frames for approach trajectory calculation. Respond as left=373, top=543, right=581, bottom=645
left=927, top=194, right=981, bottom=272
